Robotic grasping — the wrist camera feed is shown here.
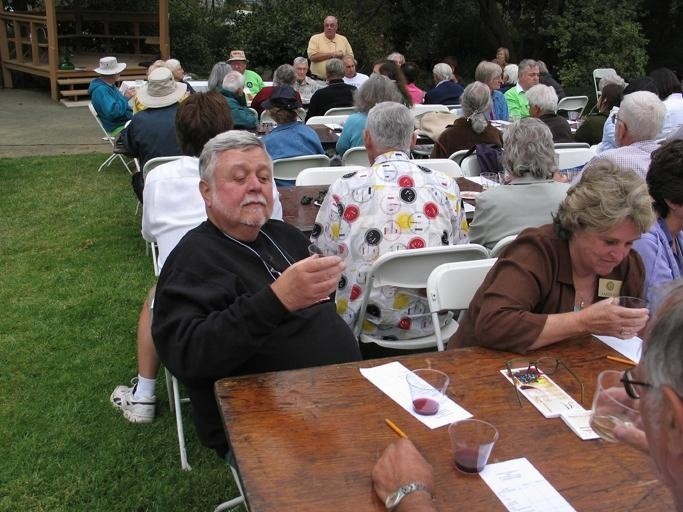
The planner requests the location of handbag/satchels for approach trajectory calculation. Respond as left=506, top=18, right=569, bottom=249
left=459, top=143, right=504, bottom=174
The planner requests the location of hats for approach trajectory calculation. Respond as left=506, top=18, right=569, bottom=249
left=137, top=68, right=188, bottom=108
left=93, top=57, right=127, bottom=76
left=226, top=50, right=246, bottom=62
left=260, top=88, right=298, bottom=109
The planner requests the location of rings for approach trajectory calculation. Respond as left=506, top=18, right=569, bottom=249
left=619, top=326, right=624, bottom=335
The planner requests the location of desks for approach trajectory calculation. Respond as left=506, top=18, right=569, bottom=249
left=212, top=312, right=678, bottom=512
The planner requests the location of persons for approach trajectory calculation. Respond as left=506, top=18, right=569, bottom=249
left=109, top=91, right=283, bottom=425
left=447, top=158, right=651, bottom=356
left=631, top=139, right=683, bottom=310
left=151, top=128, right=361, bottom=499
left=306, top=16, right=358, bottom=87
left=574, top=90, right=667, bottom=182
left=309, top=100, right=471, bottom=357
left=468, top=116, right=574, bottom=251
left=370, top=281, right=681, bottom=512
left=88, top=47, right=682, bottom=187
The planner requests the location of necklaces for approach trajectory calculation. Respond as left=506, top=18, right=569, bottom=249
left=574, top=281, right=597, bottom=309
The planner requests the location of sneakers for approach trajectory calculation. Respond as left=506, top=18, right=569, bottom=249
left=110, top=377, right=157, bottom=423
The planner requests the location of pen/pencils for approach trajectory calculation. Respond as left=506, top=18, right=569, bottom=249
left=607, top=355, right=638, bottom=366
left=385, top=419, right=409, bottom=439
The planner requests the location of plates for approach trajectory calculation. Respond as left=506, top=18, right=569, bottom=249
left=461, top=191, right=479, bottom=199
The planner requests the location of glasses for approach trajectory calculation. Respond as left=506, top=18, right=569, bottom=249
left=610, top=112, right=628, bottom=132
left=504, top=355, right=584, bottom=407
left=619, top=366, right=659, bottom=400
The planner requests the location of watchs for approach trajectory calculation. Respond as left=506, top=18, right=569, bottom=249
left=384, top=482, right=435, bottom=512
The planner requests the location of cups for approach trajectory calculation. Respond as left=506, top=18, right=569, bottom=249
left=480, top=172, right=497, bottom=188
left=406, top=369, right=449, bottom=415
left=568, top=111, right=578, bottom=120
left=611, top=296, right=648, bottom=308
left=308, top=243, right=346, bottom=258
left=589, top=369, right=641, bottom=443
left=449, top=420, right=498, bottom=476
left=498, top=171, right=507, bottom=184
